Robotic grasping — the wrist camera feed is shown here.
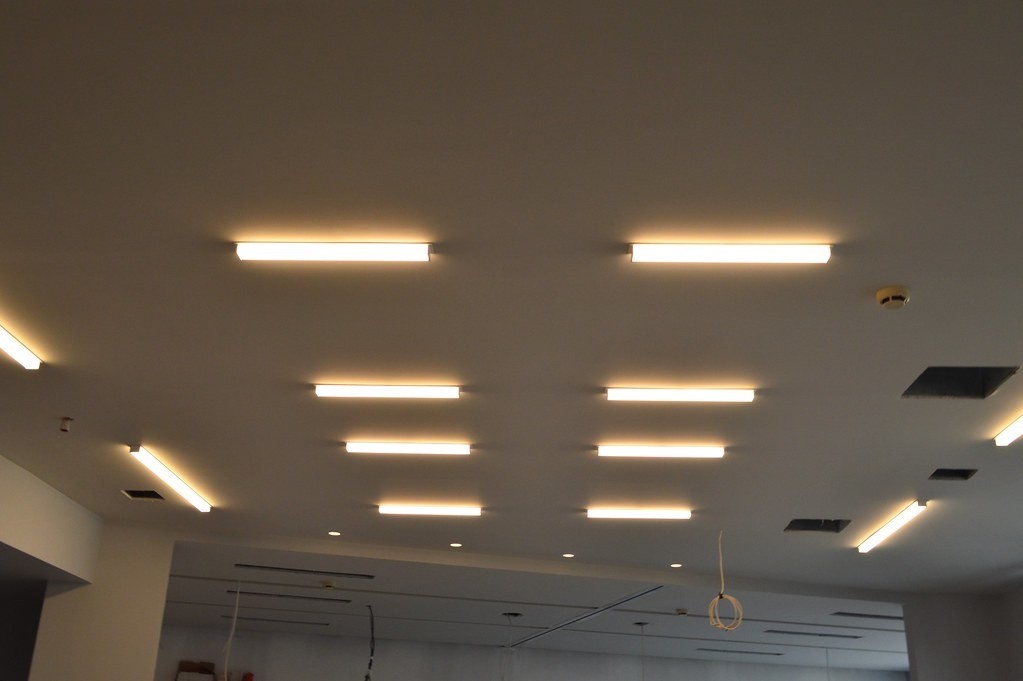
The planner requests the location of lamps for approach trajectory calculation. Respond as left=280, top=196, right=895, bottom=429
left=230, top=241, right=436, bottom=264
left=0, top=325, right=44, bottom=370
left=992, top=414, right=1023, bottom=449
left=379, top=505, right=483, bottom=517
left=312, top=383, right=462, bottom=400
left=603, top=387, right=759, bottom=406
left=344, top=442, right=472, bottom=455
left=595, top=444, right=729, bottom=462
left=585, top=505, right=696, bottom=519
left=857, top=499, right=928, bottom=554
left=129, top=444, right=211, bottom=512
left=623, top=241, right=836, bottom=266
